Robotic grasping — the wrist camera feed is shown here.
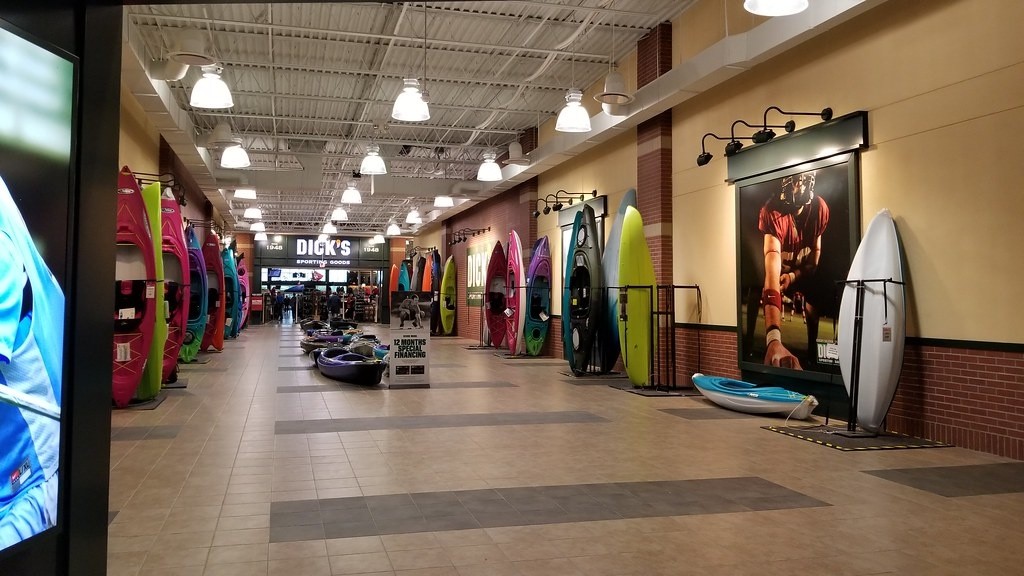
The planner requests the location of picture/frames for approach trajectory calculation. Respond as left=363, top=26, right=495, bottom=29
left=0, top=17, right=82, bottom=562
left=734, top=148, right=861, bottom=386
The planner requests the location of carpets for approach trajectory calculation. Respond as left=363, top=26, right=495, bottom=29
left=760, top=423, right=956, bottom=451
left=557, top=370, right=628, bottom=379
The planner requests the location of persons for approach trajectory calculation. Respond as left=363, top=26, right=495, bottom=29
left=399, top=294, right=425, bottom=328
left=747, top=169, right=829, bottom=370
left=347, top=293, right=356, bottom=320
left=330, top=292, right=341, bottom=317
left=272, top=292, right=303, bottom=322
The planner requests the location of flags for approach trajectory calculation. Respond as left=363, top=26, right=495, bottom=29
left=312, top=271, right=324, bottom=280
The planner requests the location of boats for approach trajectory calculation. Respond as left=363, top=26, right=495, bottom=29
left=299, top=315, right=391, bottom=372
left=110, top=164, right=158, bottom=411
left=567, top=203, right=603, bottom=377
left=219, top=247, right=251, bottom=340
left=159, top=184, right=193, bottom=384
left=485, top=241, right=508, bottom=350
left=525, top=236, right=550, bottom=356
left=318, top=346, right=388, bottom=386
left=560, top=210, right=582, bottom=374
left=616, top=205, right=658, bottom=388
left=837, top=208, right=908, bottom=433
left=439, top=254, right=457, bottom=337
left=177, top=221, right=209, bottom=363
left=202, top=228, right=225, bottom=355
left=388, top=249, right=441, bottom=334
left=601, top=189, right=638, bottom=341
left=131, top=180, right=170, bottom=403
left=503, top=229, right=527, bottom=357
left=691, top=371, right=819, bottom=419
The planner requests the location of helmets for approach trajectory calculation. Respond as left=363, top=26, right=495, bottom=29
left=781, top=170, right=816, bottom=208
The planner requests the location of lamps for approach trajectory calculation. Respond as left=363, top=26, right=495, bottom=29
left=433, top=196, right=454, bottom=207
left=254, top=232, right=268, bottom=241
left=219, top=116, right=251, bottom=168
left=341, top=179, right=363, bottom=205
left=697, top=133, right=757, bottom=166
left=390, top=2, right=430, bottom=121
left=372, top=234, right=385, bottom=244
left=742, top=0, right=809, bottom=17
left=331, top=203, right=349, bottom=221
left=552, top=189, right=597, bottom=211
left=231, top=189, right=258, bottom=200
left=184, top=217, right=223, bottom=240
left=189, top=21, right=236, bottom=108
left=386, top=218, right=402, bottom=236
left=406, top=202, right=422, bottom=224
left=533, top=198, right=573, bottom=218
left=322, top=221, right=337, bottom=234
left=752, top=106, right=833, bottom=142
left=725, top=119, right=795, bottom=157
left=360, top=132, right=387, bottom=176
left=250, top=217, right=265, bottom=231
left=542, top=193, right=584, bottom=216
left=554, top=17, right=593, bottom=133
left=410, top=246, right=436, bottom=258
left=243, top=203, right=262, bottom=219
left=133, top=171, right=188, bottom=207
left=477, top=133, right=503, bottom=182
left=448, top=227, right=490, bottom=246
